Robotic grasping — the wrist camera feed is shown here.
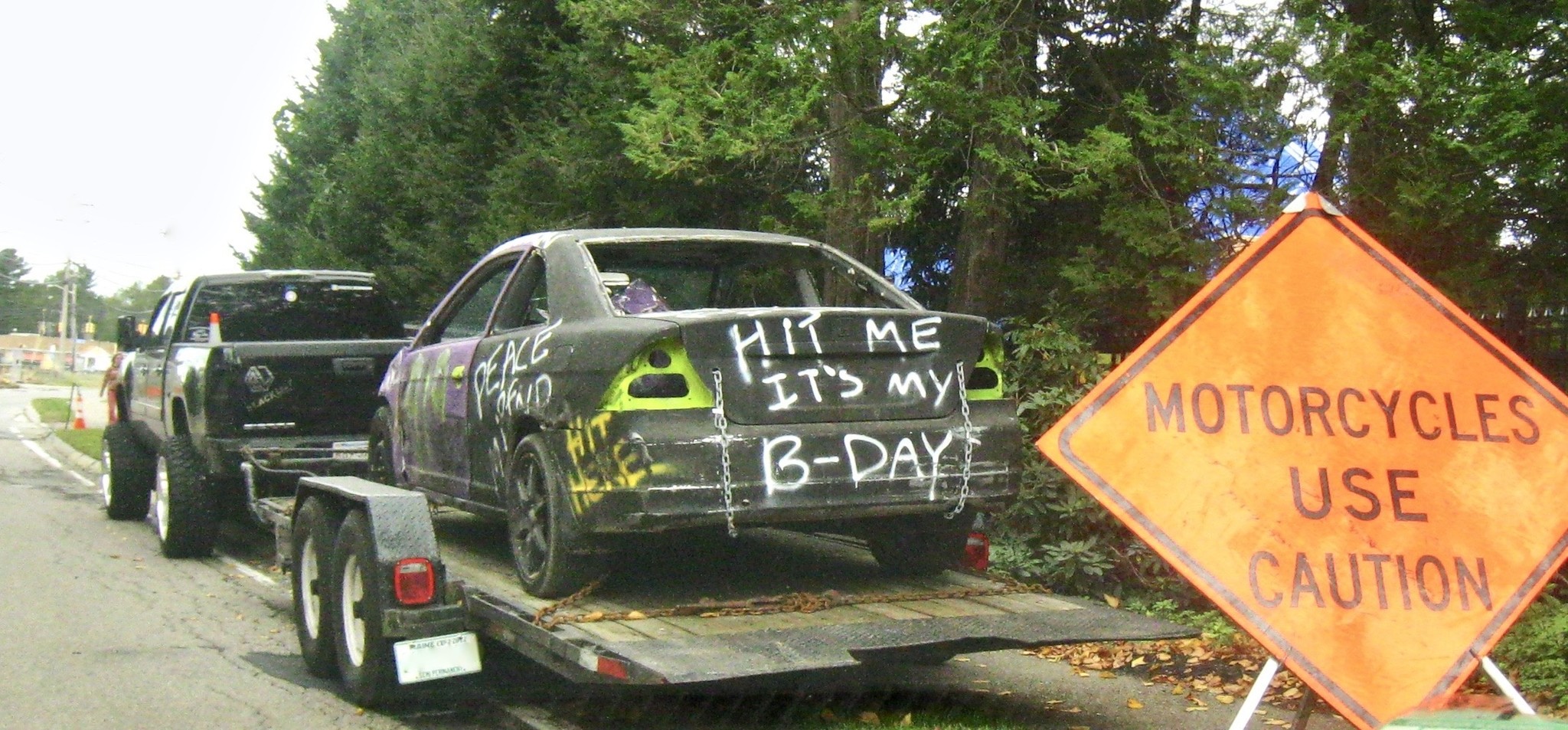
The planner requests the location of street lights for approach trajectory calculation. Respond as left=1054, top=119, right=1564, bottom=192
left=47, top=284, right=77, bottom=373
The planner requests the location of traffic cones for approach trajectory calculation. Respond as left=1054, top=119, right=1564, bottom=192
left=71, top=396, right=86, bottom=431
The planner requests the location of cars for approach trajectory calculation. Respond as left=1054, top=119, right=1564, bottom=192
left=367, top=224, right=1026, bottom=602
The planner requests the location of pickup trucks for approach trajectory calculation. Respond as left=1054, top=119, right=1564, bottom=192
left=101, top=268, right=420, bottom=561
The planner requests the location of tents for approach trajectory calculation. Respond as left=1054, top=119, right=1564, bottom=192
left=75, top=346, right=113, bottom=371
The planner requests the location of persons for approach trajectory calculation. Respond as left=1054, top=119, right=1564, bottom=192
left=99, top=353, right=124, bottom=421
